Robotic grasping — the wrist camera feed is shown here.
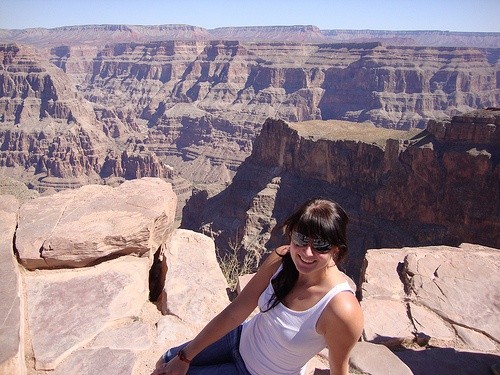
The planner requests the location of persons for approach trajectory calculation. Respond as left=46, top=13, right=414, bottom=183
left=146, top=197, right=366, bottom=375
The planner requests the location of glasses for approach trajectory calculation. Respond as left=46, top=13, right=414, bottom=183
left=289, top=229, right=331, bottom=255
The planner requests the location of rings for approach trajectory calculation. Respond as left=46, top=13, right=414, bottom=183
left=157, top=363, right=171, bottom=374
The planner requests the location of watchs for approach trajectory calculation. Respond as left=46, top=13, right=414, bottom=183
left=178, top=348, right=191, bottom=364
